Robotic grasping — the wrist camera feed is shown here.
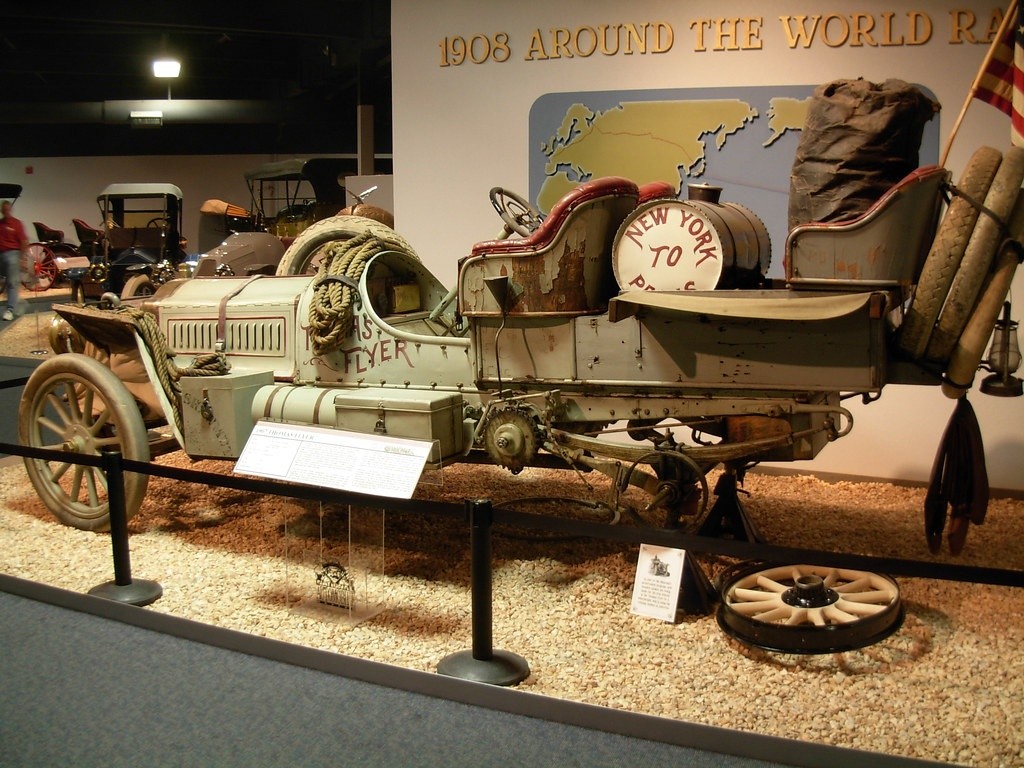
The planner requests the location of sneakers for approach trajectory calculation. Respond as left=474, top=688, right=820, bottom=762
left=3, top=310, right=14, bottom=320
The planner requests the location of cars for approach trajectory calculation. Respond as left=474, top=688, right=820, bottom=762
left=75, top=183, right=190, bottom=303
left=19, top=161, right=912, bottom=533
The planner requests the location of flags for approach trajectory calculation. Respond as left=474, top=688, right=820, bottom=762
left=969, top=0, right=1024, bottom=150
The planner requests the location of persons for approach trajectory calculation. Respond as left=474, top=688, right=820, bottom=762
left=0, top=200, right=29, bottom=320
